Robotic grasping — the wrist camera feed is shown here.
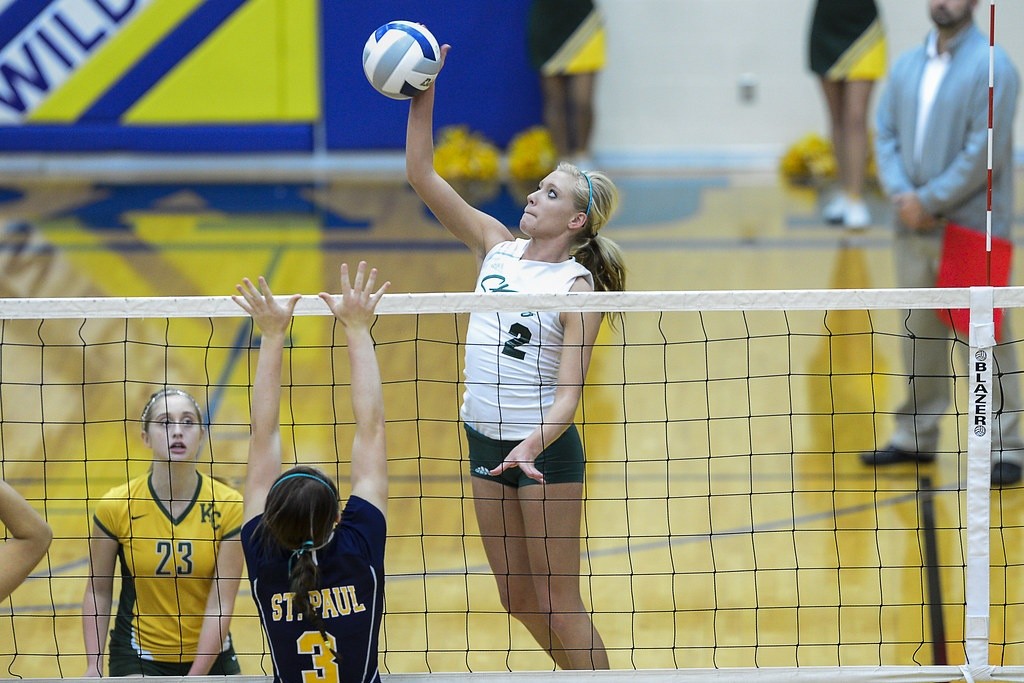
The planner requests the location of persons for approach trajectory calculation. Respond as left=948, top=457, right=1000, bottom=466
left=232, top=261, right=392, bottom=683
left=405, top=22, right=626, bottom=671
left=83, top=388, right=244, bottom=677
left=809, top=0, right=887, bottom=228
left=526, top=0, right=605, bottom=171
left=0, top=478, right=53, bottom=602
left=860, top=0, right=1023, bottom=485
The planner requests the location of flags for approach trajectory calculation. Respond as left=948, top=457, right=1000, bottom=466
left=934, top=224, right=1013, bottom=342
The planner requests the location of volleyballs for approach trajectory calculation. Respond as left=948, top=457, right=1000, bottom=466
left=362, top=19, right=444, bottom=100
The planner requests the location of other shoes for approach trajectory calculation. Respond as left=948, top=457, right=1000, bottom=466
left=571, top=154, right=592, bottom=173
left=861, top=446, right=934, bottom=465
left=991, top=462, right=1021, bottom=486
left=825, top=194, right=870, bottom=230
